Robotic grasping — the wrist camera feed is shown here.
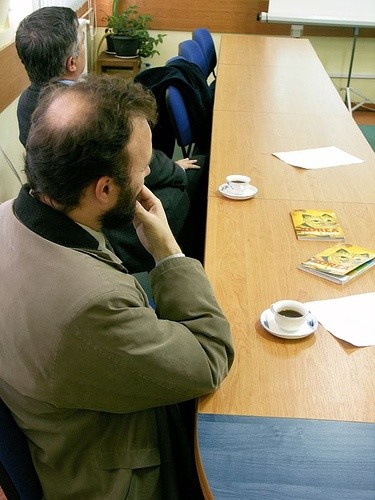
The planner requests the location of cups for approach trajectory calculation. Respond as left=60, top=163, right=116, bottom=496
left=272, top=300, right=308, bottom=331
left=225, top=175, right=251, bottom=195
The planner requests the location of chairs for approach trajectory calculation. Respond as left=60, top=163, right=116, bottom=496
left=133, top=29, right=218, bottom=160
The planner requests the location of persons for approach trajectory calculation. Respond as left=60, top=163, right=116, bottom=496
left=15, top=6, right=209, bottom=272
left=0, top=74, right=235, bottom=500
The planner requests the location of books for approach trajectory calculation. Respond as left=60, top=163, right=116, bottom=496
left=290, top=209, right=345, bottom=242
left=297, top=242, right=375, bottom=285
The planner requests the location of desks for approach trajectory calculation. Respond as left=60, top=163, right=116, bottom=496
left=97, top=51, right=141, bottom=76
left=193, top=32, right=375, bottom=500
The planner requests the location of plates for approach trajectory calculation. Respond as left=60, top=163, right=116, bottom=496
left=260, top=308, right=318, bottom=339
left=218, top=183, right=257, bottom=200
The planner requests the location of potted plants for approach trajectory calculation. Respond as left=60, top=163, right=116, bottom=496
left=98, top=0, right=160, bottom=60
